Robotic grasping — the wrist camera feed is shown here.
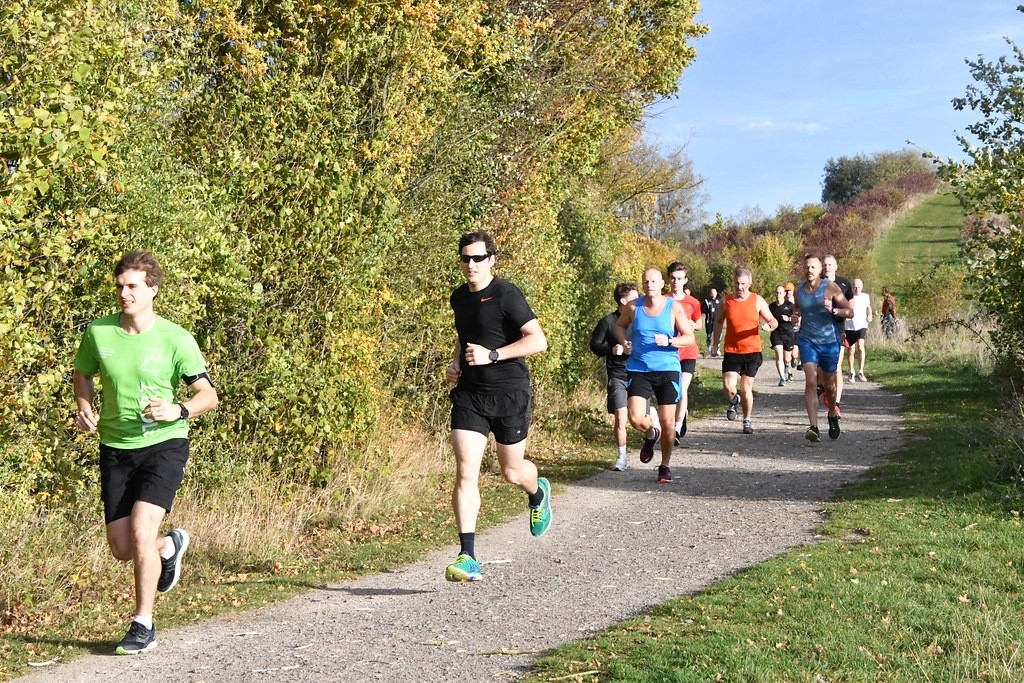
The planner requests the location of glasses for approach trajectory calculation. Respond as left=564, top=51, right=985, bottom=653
left=460, top=254, right=489, bottom=263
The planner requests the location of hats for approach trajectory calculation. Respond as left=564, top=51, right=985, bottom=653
left=784, top=282, right=794, bottom=291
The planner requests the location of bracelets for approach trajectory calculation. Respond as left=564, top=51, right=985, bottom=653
left=768, top=323, right=772, bottom=329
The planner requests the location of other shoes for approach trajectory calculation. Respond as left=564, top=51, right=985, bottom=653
left=848, top=372, right=856, bottom=383
left=716, top=350, right=721, bottom=356
left=707, top=349, right=712, bottom=357
left=858, top=372, right=867, bottom=382
left=778, top=359, right=840, bottom=407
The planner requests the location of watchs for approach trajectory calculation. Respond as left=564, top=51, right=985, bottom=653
left=831, top=307, right=835, bottom=314
left=178, top=403, right=189, bottom=420
left=666, top=334, right=672, bottom=347
left=490, top=348, right=499, bottom=364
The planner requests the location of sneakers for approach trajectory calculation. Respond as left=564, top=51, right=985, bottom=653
left=805, top=425, right=821, bottom=443
left=640, top=427, right=661, bottom=463
left=112, top=621, right=158, bottom=655
left=650, top=407, right=661, bottom=435
left=674, top=431, right=680, bottom=446
left=611, top=457, right=628, bottom=472
left=528, top=477, right=552, bottom=538
left=653, top=465, right=673, bottom=483
left=445, top=551, right=483, bottom=582
left=157, top=529, right=190, bottom=593
left=680, top=411, right=688, bottom=437
left=743, top=420, right=753, bottom=434
left=828, top=412, right=841, bottom=440
left=726, top=394, right=741, bottom=420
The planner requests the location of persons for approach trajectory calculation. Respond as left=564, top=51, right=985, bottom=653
left=442, top=230, right=552, bottom=583
left=844, top=278, right=874, bottom=382
left=879, top=286, right=897, bottom=334
left=790, top=254, right=854, bottom=443
left=700, top=289, right=722, bottom=357
left=767, top=285, right=795, bottom=388
left=71, top=251, right=219, bottom=656
left=711, top=268, right=779, bottom=434
left=662, top=262, right=703, bottom=447
left=614, top=267, right=695, bottom=483
left=591, top=282, right=659, bottom=471
left=784, top=282, right=802, bottom=371
left=815, top=254, right=854, bottom=420
